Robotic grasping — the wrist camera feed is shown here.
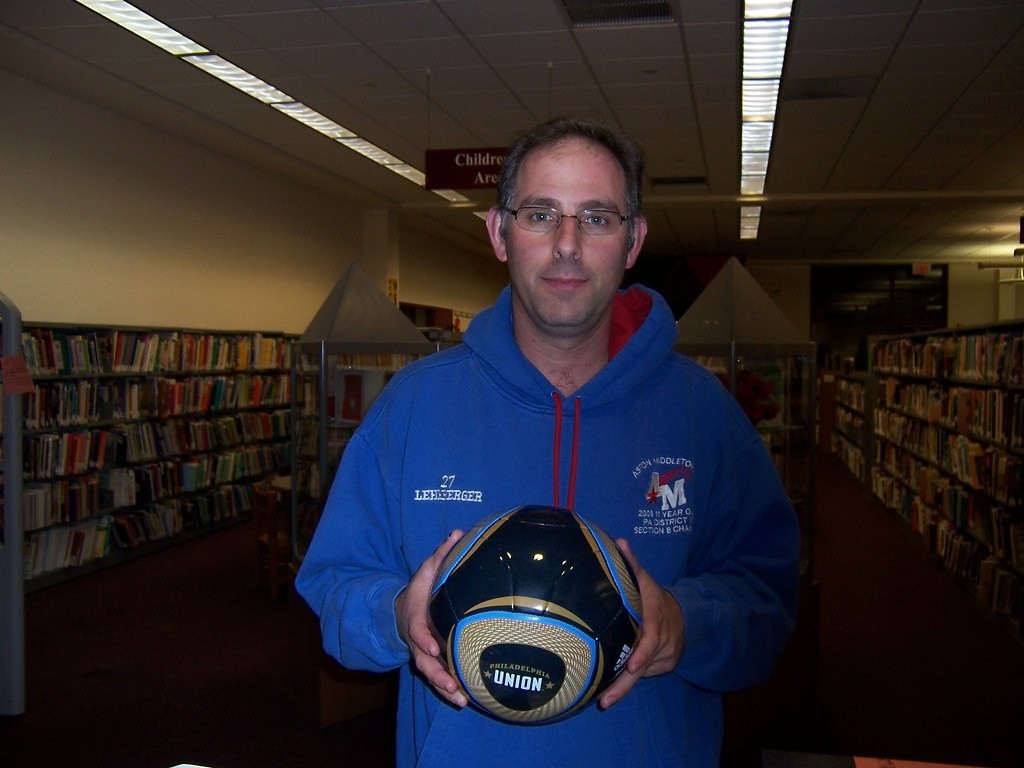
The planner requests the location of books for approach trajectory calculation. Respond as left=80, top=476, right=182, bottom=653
left=684, top=354, right=787, bottom=469
left=816, top=329, right=1024, bottom=636
left=0, top=329, right=427, bottom=580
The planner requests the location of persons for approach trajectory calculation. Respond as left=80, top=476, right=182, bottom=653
left=294, top=117, right=802, bottom=768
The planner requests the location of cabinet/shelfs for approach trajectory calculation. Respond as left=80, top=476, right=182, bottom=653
left=284, top=339, right=456, bottom=575
left=0, top=319, right=444, bottom=595
left=831, top=375, right=866, bottom=491
left=866, top=317, right=1024, bottom=649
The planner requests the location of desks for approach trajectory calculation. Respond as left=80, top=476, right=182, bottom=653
left=673, top=341, right=821, bottom=752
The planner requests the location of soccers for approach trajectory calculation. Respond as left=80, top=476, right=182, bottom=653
left=420, top=507, right=646, bottom=725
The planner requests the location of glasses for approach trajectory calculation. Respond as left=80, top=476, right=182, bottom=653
left=499, top=205, right=628, bottom=236
